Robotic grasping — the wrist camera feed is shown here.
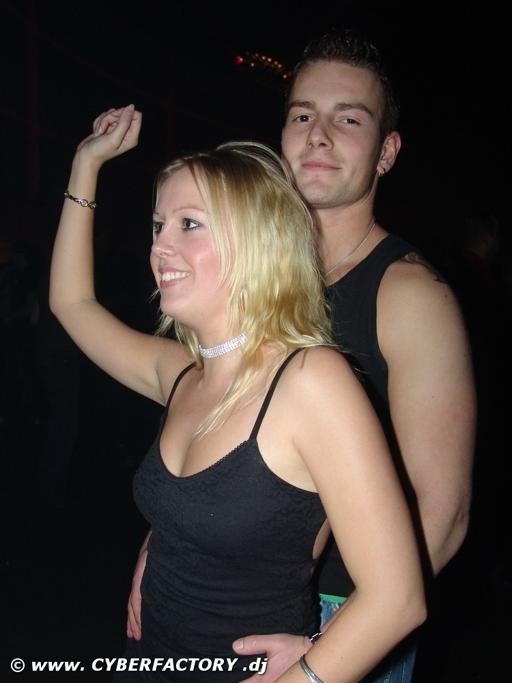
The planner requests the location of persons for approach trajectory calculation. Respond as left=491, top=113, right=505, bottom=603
left=48, top=101, right=430, bottom=682
left=125, top=27, right=479, bottom=682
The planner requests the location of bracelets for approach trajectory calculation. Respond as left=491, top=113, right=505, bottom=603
left=62, top=187, right=98, bottom=209
left=298, top=653, right=325, bottom=682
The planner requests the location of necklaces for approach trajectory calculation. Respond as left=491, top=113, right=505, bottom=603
left=198, top=329, right=248, bottom=359
left=323, top=220, right=378, bottom=279
